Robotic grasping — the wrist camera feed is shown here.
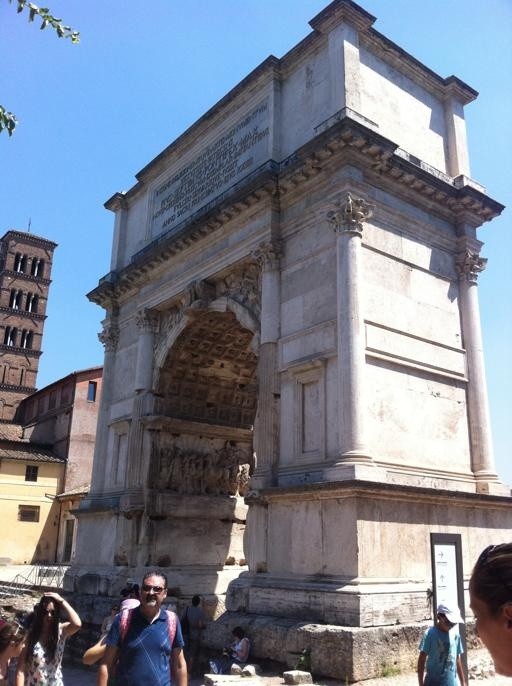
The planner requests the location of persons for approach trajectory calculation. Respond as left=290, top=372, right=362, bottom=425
left=81, top=597, right=141, bottom=666
left=180, top=593, right=207, bottom=680
left=0, top=618, right=28, bottom=686
left=207, top=625, right=251, bottom=675
left=98, top=569, right=189, bottom=686
left=100, top=604, right=119, bottom=635
left=416, top=599, right=467, bottom=686
left=14, top=591, right=83, bottom=686
left=469, top=541, right=512, bottom=679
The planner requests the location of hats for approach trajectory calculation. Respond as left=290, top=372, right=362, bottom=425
left=437, top=602, right=465, bottom=624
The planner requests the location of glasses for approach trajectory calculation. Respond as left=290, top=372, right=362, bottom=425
left=143, top=584, right=163, bottom=592
